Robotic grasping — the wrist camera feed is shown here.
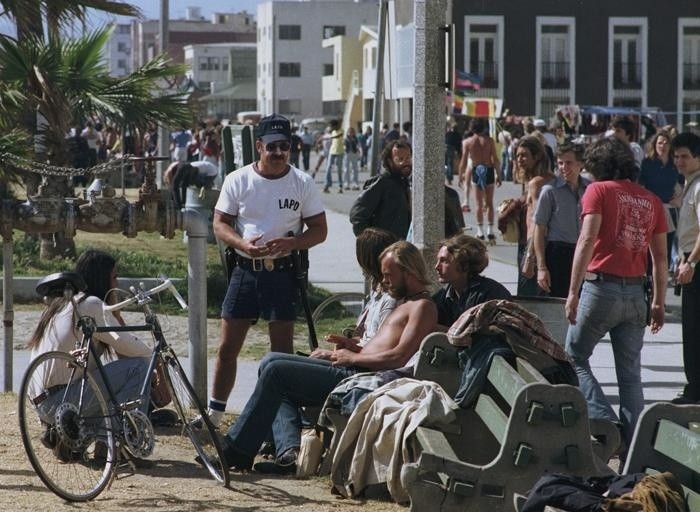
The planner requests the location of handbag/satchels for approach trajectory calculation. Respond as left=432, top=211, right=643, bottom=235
left=546, top=246, right=565, bottom=270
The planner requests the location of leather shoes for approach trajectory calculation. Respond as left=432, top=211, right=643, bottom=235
left=671, top=394, right=696, bottom=405
left=94, top=443, right=151, bottom=466
left=42, top=429, right=76, bottom=463
left=253, top=458, right=299, bottom=475
left=196, top=455, right=245, bottom=471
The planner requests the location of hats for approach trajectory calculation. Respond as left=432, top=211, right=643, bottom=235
left=257, top=112, right=291, bottom=144
left=329, top=130, right=344, bottom=138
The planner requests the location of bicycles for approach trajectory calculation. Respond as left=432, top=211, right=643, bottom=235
left=19, top=273, right=230, bottom=501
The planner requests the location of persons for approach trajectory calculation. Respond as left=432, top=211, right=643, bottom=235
left=164, top=161, right=218, bottom=212
left=189, top=113, right=328, bottom=430
left=461, top=131, right=487, bottom=213
left=430, top=235, right=514, bottom=333
left=498, top=136, right=557, bottom=298
left=298, top=125, right=314, bottom=170
left=195, top=241, right=438, bottom=474
left=499, top=124, right=513, bottom=181
left=186, top=122, right=223, bottom=161
left=446, top=116, right=462, bottom=184
left=660, top=125, right=679, bottom=140
left=566, top=137, right=669, bottom=475
left=355, top=129, right=363, bottom=160
left=507, top=133, right=521, bottom=183
left=344, top=127, right=360, bottom=190
left=561, top=108, right=580, bottom=136
left=361, top=126, right=373, bottom=167
left=556, top=126, right=566, bottom=145
left=324, top=129, right=343, bottom=193
left=531, top=130, right=551, bottom=173
left=458, top=117, right=502, bottom=240
left=534, top=119, right=556, bottom=159
left=65, top=121, right=116, bottom=187
left=445, top=183, right=465, bottom=239
left=326, top=227, right=401, bottom=354
left=311, top=127, right=332, bottom=180
left=637, top=130, right=681, bottom=290
left=349, top=140, right=412, bottom=311
left=289, top=128, right=304, bottom=169
left=533, top=143, right=593, bottom=389
left=671, top=132, right=700, bottom=405
left=126, top=124, right=157, bottom=177
left=522, top=124, right=537, bottom=140
left=607, top=115, right=645, bottom=172
left=398, top=122, right=411, bottom=146
left=170, top=129, right=192, bottom=162
left=366, top=134, right=373, bottom=171
left=383, top=123, right=400, bottom=150
left=27, top=249, right=173, bottom=462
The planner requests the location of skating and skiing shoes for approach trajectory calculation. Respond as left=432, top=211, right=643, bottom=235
left=474, top=233, right=484, bottom=244
left=489, top=234, right=495, bottom=244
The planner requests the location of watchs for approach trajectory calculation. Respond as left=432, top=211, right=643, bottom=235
left=685, top=260, right=697, bottom=268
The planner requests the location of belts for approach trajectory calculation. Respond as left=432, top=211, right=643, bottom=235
left=517, top=245, right=527, bottom=253
left=25, top=384, right=67, bottom=405
left=584, top=271, right=647, bottom=286
left=236, top=255, right=296, bottom=272
left=683, top=253, right=692, bottom=262
left=551, top=242, right=578, bottom=250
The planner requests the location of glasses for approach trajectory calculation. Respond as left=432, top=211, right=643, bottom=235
left=260, top=143, right=291, bottom=151
left=391, top=156, right=411, bottom=164
left=657, top=140, right=669, bottom=146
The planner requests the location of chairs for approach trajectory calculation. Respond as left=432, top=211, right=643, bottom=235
left=614, top=380, right=700, bottom=512
left=378, top=333, right=541, bottom=512
left=485, top=312, right=624, bottom=512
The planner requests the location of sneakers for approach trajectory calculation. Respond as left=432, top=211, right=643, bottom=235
left=183, top=412, right=220, bottom=433
